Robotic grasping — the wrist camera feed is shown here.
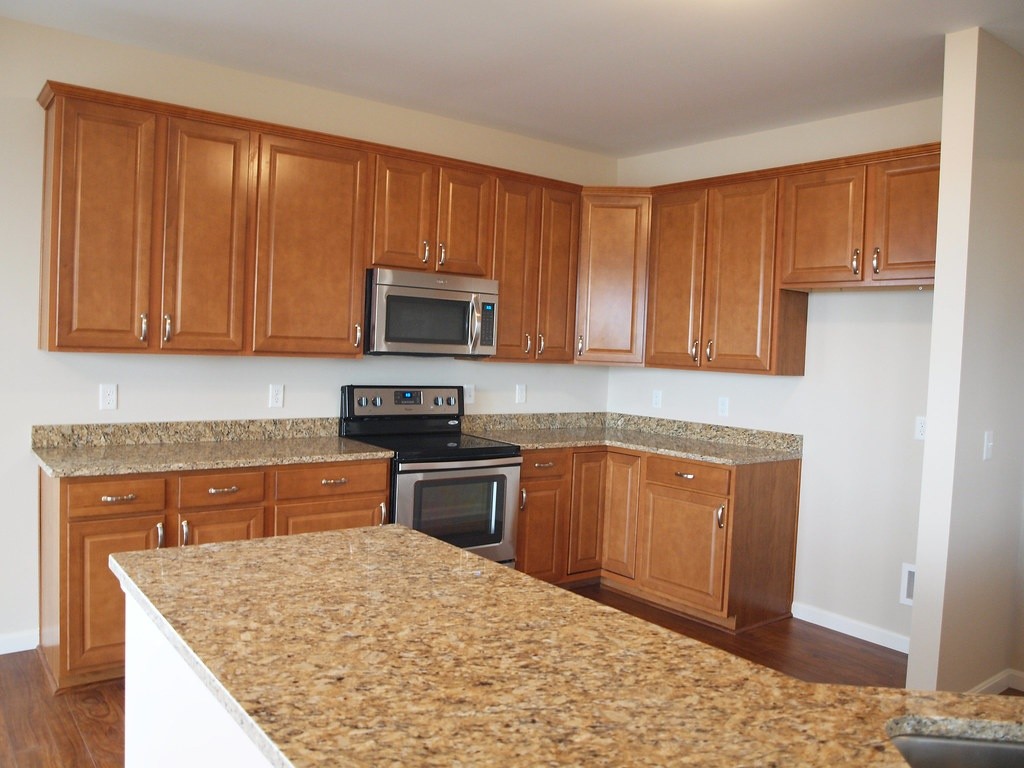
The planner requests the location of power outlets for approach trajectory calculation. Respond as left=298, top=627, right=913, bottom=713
left=516, top=384, right=527, bottom=404
left=464, top=385, right=475, bottom=404
left=269, top=384, right=285, bottom=408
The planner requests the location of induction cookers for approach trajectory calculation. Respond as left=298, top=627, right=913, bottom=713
left=339, top=384, right=520, bottom=461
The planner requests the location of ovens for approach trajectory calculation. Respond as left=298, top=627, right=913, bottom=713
left=391, top=456, right=523, bottom=568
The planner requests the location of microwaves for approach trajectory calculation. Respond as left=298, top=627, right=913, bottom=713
left=362, top=268, right=499, bottom=357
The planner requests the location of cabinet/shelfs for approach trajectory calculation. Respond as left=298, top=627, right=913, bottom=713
left=779, top=139, right=940, bottom=293
left=453, top=166, right=583, bottom=366
left=464, top=412, right=606, bottom=589
left=649, top=167, right=809, bottom=378
left=29, top=417, right=268, bottom=697
left=109, top=521, right=1024, bottom=768
left=599, top=431, right=804, bottom=636
left=249, top=119, right=373, bottom=362
left=225, top=418, right=396, bottom=536
left=573, top=184, right=652, bottom=368
left=369, top=141, right=493, bottom=278
left=36, top=80, right=254, bottom=360
